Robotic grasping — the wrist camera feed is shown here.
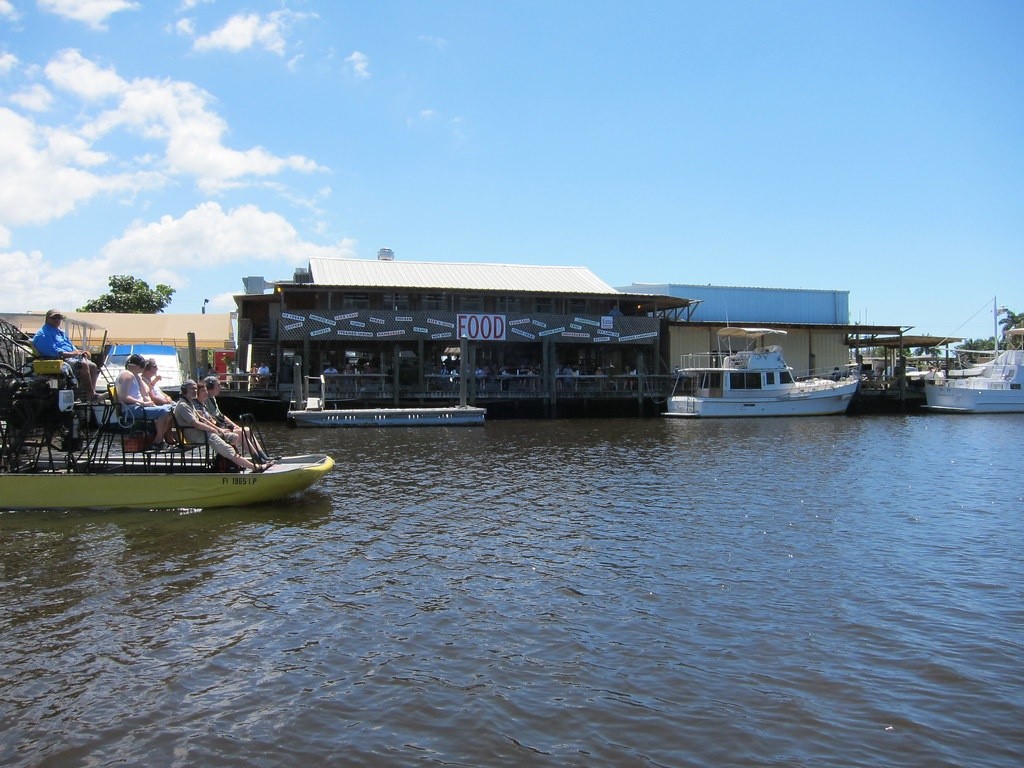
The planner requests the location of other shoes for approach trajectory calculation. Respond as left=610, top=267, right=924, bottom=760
left=256, top=456, right=282, bottom=463
left=150, top=439, right=176, bottom=450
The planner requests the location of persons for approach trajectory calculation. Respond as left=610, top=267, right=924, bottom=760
left=555, top=363, right=586, bottom=393
left=116, top=354, right=182, bottom=452
left=608, top=304, right=623, bottom=317
left=516, top=364, right=530, bottom=389
left=441, top=366, right=449, bottom=375
left=195, top=362, right=204, bottom=382
left=191, top=383, right=240, bottom=472
left=255, top=363, right=271, bottom=385
left=31, top=309, right=108, bottom=405
left=475, top=366, right=508, bottom=390
left=623, top=366, right=633, bottom=390
left=528, top=365, right=539, bottom=391
left=342, top=364, right=353, bottom=374
left=249, top=364, right=259, bottom=391
left=173, top=379, right=275, bottom=473
left=323, top=366, right=338, bottom=373
left=594, top=367, right=605, bottom=391
left=203, top=376, right=275, bottom=465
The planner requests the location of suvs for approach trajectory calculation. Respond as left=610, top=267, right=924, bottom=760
left=882, top=365, right=924, bottom=387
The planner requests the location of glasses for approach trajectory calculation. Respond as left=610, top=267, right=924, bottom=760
left=153, top=364, right=157, bottom=368
left=50, top=317, right=62, bottom=320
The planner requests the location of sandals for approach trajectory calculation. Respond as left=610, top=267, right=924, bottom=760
left=253, top=462, right=274, bottom=473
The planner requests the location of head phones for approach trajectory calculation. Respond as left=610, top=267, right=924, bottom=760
left=181, top=381, right=187, bottom=394
left=209, top=378, right=214, bottom=390
left=145, top=359, right=153, bottom=370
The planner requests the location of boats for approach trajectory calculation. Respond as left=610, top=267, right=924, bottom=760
left=91, top=341, right=184, bottom=429
left=922, top=293, right=1024, bottom=413
left=0, top=450, right=334, bottom=509
left=659, top=327, right=858, bottom=419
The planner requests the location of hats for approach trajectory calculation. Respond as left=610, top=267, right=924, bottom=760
left=46, top=309, right=62, bottom=317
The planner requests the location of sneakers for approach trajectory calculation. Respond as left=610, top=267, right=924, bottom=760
left=89, top=392, right=109, bottom=404
